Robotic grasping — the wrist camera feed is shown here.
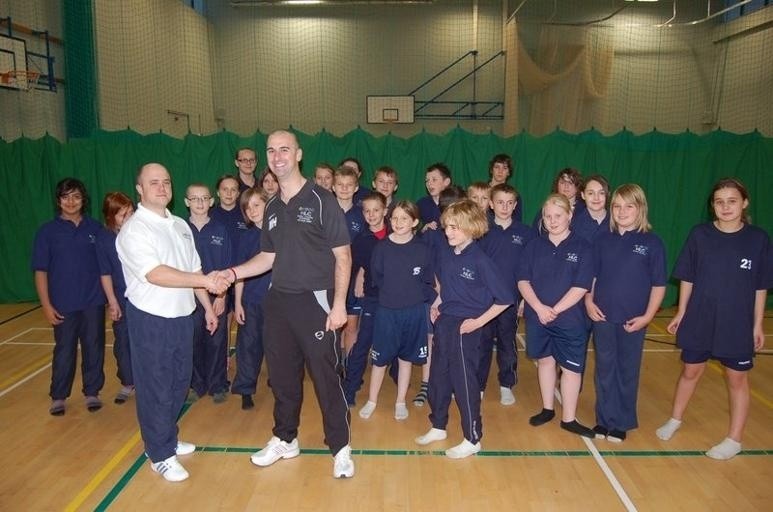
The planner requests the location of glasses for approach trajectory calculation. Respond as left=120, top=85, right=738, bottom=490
left=237, top=158, right=258, bottom=163
left=186, top=196, right=213, bottom=200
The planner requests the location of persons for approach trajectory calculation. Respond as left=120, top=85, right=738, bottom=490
left=102, top=190, right=134, bottom=403
left=178, top=148, right=280, bottom=409
left=316, top=152, right=531, bottom=458
left=115, top=163, right=232, bottom=483
left=655, top=179, right=772, bottom=460
left=31, top=178, right=122, bottom=415
left=207, top=130, right=353, bottom=479
left=516, top=168, right=667, bottom=441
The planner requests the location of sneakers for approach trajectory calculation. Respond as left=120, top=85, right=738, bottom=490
left=146, top=437, right=196, bottom=456
left=251, top=437, right=300, bottom=466
left=150, top=455, right=189, bottom=482
left=333, top=446, right=354, bottom=478
